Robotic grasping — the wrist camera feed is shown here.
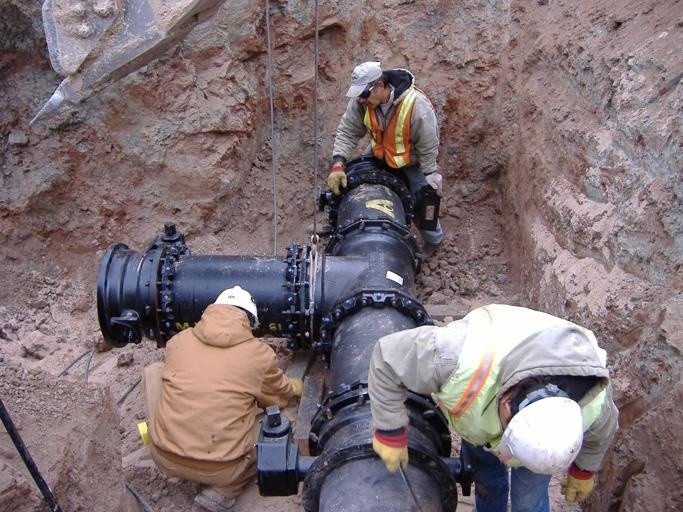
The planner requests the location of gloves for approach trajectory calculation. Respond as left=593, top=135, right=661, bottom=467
left=560, top=462, right=595, bottom=503
left=372, top=429, right=408, bottom=472
left=290, top=378, right=303, bottom=397
left=426, top=171, right=444, bottom=195
left=327, top=167, right=347, bottom=195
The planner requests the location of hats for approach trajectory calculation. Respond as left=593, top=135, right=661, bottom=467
left=346, top=61, right=382, bottom=98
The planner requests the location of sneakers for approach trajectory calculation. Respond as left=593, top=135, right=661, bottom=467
left=194, top=488, right=236, bottom=511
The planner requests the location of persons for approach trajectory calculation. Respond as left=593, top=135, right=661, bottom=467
left=365, top=302, right=622, bottom=512
left=325, top=60, right=446, bottom=265
left=139, top=284, right=305, bottom=512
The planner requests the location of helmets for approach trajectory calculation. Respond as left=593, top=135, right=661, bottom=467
left=214, top=285, right=259, bottom=330
left=497, top=396, right=583, bottom=475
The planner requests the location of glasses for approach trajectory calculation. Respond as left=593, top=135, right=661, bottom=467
left=358, top=86, right=376, bottom=99
